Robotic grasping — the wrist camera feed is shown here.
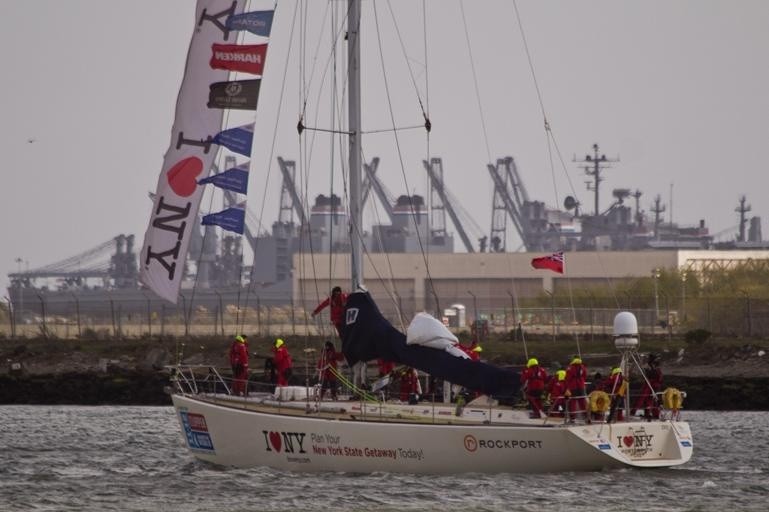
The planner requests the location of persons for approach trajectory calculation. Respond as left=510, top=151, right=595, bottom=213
left=518, top=356, right=663, bottom=426
left=316, top=342, right=340, bottom=401
left=228, top=335, right=252, bottom=396
left=376, top=358, right=393, bottom=402
left=269, top=339, right=292, bottom=395
left=311, top=286, right=349, bottom=342
left=397, top=367, right=418, bottom=403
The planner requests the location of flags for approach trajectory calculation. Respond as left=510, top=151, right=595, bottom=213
left=531, top=251, right=565, bottom=274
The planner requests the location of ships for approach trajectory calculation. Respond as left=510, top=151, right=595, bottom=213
left=7, top=143, right=768, bottom=317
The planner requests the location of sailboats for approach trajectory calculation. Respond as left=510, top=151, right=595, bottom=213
left=138, top=0, right=693, bottom=467
